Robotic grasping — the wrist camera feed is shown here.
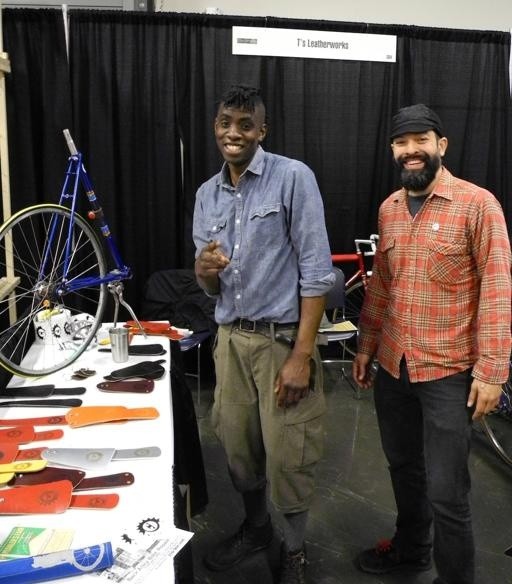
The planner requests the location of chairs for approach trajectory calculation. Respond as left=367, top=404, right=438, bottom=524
left=316, top=264, right=361, bottom=400
left=141, top=269, right=217, bottom=404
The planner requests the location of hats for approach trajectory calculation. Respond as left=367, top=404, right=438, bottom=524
left=391, top=103, right=443, bottom=139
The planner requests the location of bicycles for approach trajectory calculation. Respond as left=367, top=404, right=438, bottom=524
left=479, top=361, right=512, bottom=466
left=331, top=234, right=379, bottom=363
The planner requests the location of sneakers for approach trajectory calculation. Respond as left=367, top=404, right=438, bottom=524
left=278, top=534, right=307, bottom=584
left=206, top=513, right=274, bottom=570
left=359, top=534, right=433, bottom=572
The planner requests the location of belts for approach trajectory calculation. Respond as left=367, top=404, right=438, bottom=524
left=232, top=318, right=298, bottom=348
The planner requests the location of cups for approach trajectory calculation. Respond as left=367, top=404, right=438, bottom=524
left=109, top=328, right=129, bottom=362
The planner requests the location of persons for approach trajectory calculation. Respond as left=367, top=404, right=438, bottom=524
left=192, top=84, right=336, bottom=584
left=350, top=103, right=512, bottom=584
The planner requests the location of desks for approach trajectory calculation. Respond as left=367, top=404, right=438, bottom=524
left=0, top=320, right=174, bottom=584
